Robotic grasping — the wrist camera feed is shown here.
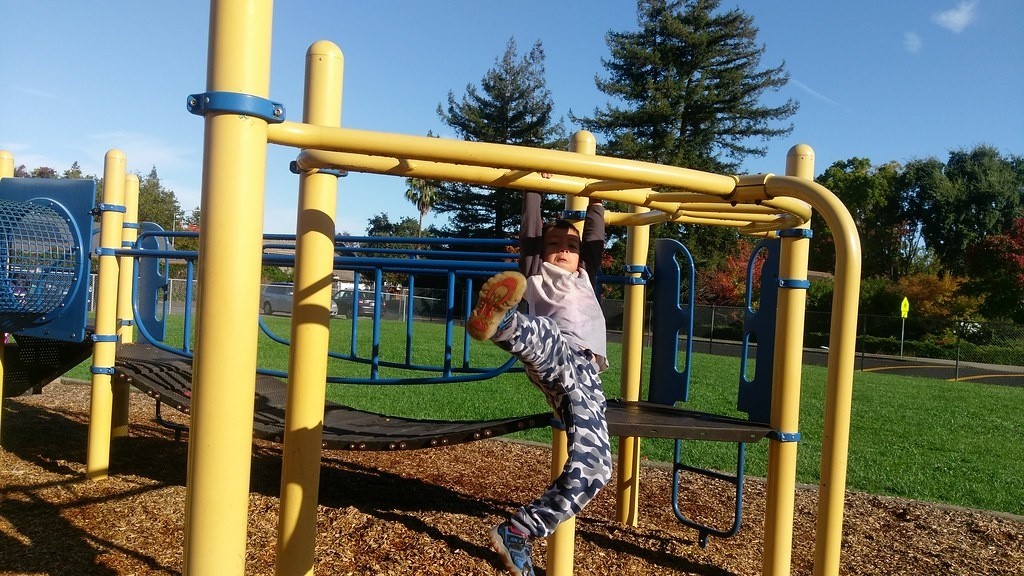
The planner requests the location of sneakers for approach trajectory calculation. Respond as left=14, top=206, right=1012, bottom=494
left=488, top=522, right=535, bottom=576
left=466, top=271, right=528, bottom=341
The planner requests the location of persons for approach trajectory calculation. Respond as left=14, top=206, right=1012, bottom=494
left=461, top=170, right=614, bottom=576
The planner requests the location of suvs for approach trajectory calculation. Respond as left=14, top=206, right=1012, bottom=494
left=259, top=282, right=338, bottom=320
left=330, top=288, right=386, bottom=321
